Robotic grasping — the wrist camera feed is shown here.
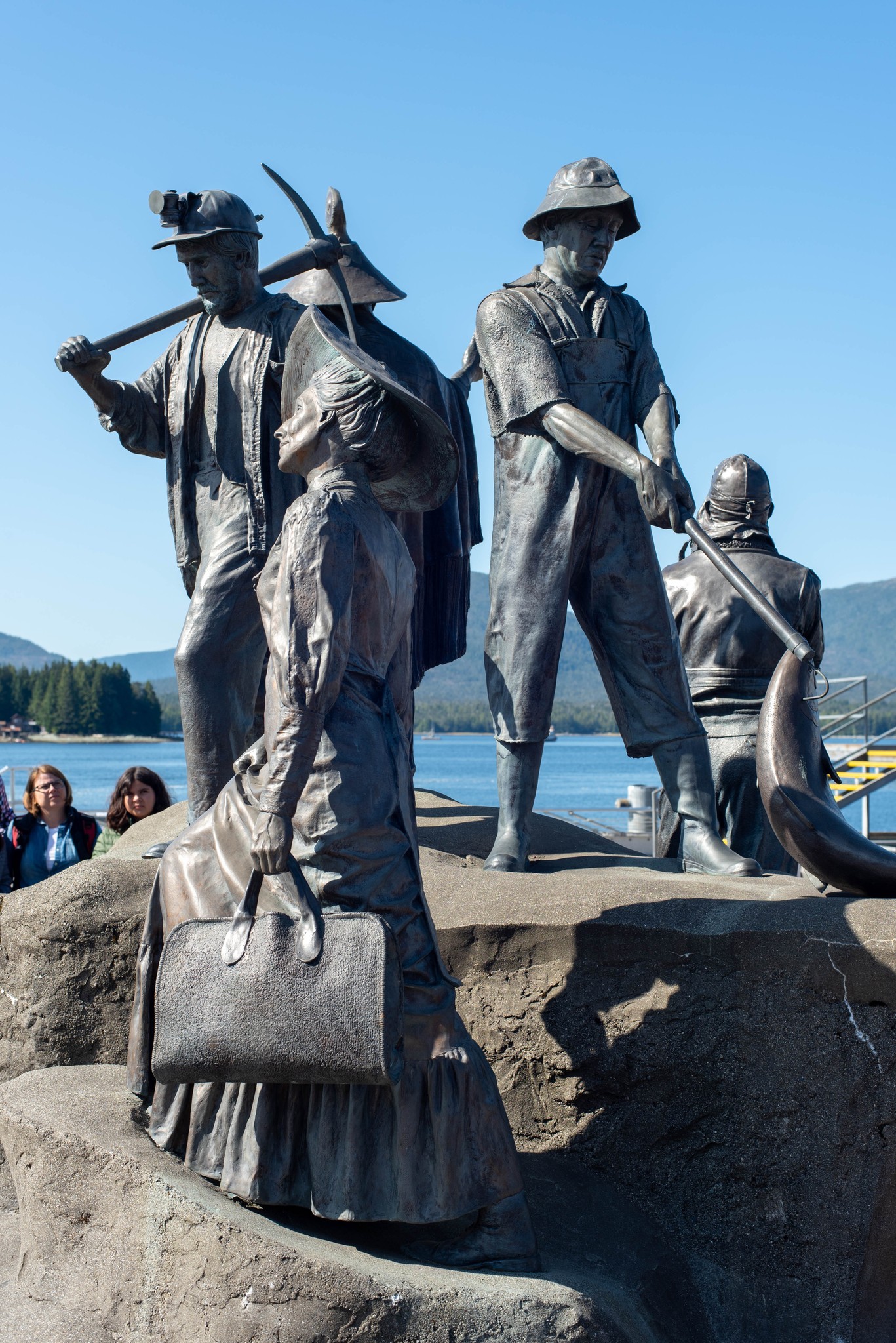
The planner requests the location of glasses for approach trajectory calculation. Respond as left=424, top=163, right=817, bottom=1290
left=31, top=780, right=64, bottom=793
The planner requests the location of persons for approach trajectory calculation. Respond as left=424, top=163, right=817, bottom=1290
left=284, top=238, right=487, bottom=698
left=90, top=767, right=172, bottom=859
left=0, top=775, right=17, bottom=894
left=475, top=163, right=764, bottom=878
left=159, top=351, right=548, bottom=1274
left=55, top=190, right=316, bottom=823
left=5, top=764, right=103, bottom=894
left=661, top=454, right=827, bottom=874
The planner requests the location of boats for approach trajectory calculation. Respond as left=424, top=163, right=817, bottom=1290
left=422, top=722, right=440, bottom=740
left=544, top=725, right=557, bottom=741
